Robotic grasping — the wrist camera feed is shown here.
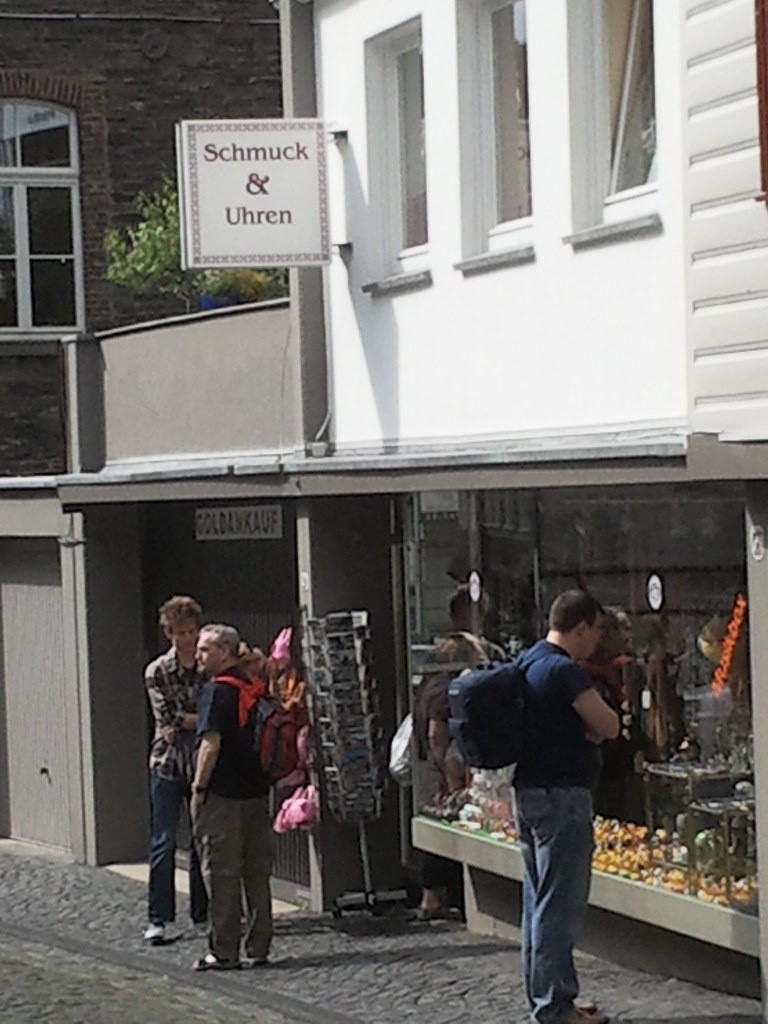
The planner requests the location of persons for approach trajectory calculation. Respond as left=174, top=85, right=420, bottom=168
left=413, top=585, right=749, bottom=828
left=190, top=625, right=276, bottom=972
left=417, top=853, right=463, bottom=920
left=510, top=590, right=619, bottom=1024
left=145, top=596, right=209, bottom=942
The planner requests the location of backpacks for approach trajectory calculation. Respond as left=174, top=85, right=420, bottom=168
left=447, top=649, right=568, bottom=770
left=216, top=671, right=298, bottom=781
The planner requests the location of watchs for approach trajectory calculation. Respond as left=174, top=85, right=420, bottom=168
left=191, top=783, right=207, bottom=794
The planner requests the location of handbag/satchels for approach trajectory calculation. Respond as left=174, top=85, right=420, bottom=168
left=271, top=786, right=319, bottom=832
left=390, top=712, right=412, bottom=787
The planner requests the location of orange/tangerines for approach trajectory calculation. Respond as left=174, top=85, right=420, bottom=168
left=590, top=818, right=756, bottom=905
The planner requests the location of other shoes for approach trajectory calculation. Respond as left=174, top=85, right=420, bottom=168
left=566, top=1009, right=610, bottom=1024
left=578, top=1002, right=598, bottom=1015
left=417, top=905, right=457, bottom=921
left=192, top=922, right=208, bottom=938
left=239, top=953, right=266, bottom=964
left=145, top=923, right=165, bottom=938
left=192, top=954, right=239, bottom=970
